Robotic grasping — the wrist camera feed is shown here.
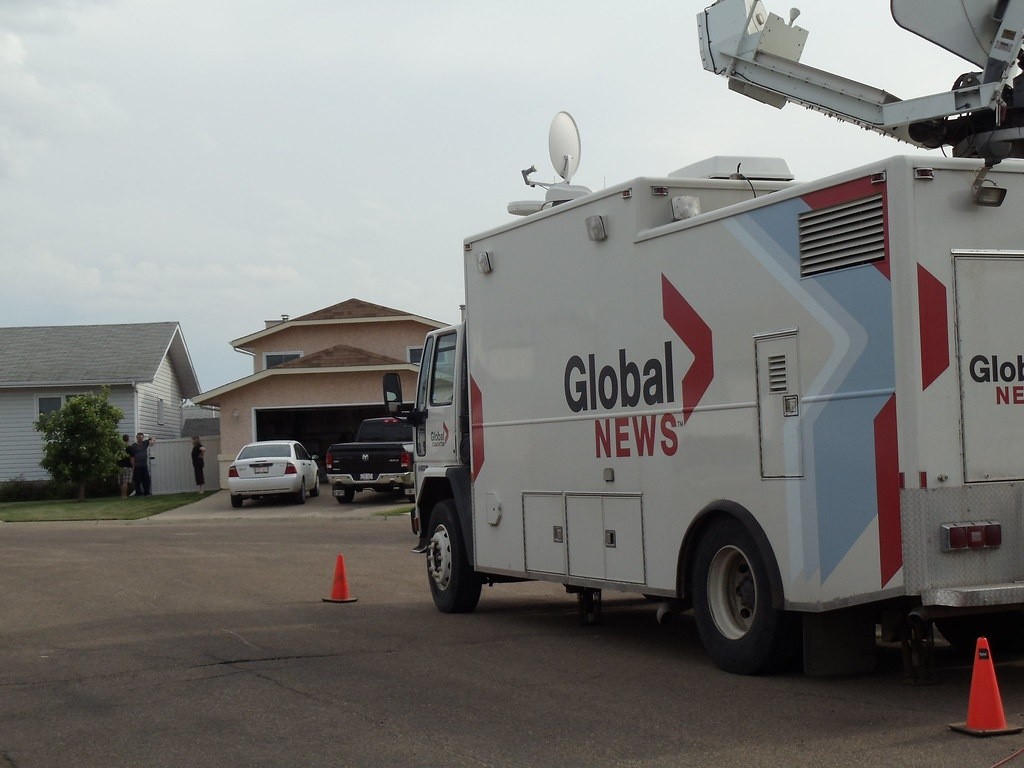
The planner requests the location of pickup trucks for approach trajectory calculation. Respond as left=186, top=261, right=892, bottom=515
left=325, top=412, right=415, bottom=504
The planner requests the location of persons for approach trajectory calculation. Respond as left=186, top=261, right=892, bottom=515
left=191, top=436, right=206, bottom=494
left=116, top=435, right=134, bottom=499
left=131, top=432, right=155, bottom=496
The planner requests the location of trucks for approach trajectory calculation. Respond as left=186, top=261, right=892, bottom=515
left=415, top=155, right=1024, bottom=675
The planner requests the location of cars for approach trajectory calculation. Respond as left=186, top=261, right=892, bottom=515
left=227, top=440, right=319, bottom=508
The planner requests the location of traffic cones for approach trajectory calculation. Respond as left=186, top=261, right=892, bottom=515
left=321, top=553, right=358, bottom=603
left=949, top=637, right=1024, bottom=738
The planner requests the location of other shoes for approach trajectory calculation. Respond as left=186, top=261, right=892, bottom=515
left=143, top=493, right=151, bottom=496
left=135, top=492, right=141, bottom=496
